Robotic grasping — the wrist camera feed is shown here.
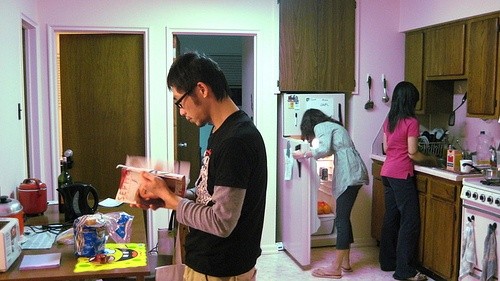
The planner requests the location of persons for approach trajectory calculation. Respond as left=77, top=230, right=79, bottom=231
left=136, top=184, right=166, bottom=207
left=141, top=52, right=267, bottom=281
left=292, top=109, right=368, bottom=279
left=380, top=81, right=439, bottom=281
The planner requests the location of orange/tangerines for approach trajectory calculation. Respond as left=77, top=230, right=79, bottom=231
left=318, top=202, right=331, bottom=214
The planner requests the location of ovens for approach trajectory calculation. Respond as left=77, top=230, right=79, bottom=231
left=459, top=199, right=500, bottom=281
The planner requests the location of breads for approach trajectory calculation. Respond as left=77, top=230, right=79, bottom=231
left=85, top=218, right=97, bottom=226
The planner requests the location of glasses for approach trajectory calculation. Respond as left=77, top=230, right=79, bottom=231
left=174, top=81, right=206, bottom=108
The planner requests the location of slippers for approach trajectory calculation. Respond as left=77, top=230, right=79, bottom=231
left=332, top=261, right=353, bottom=272
left=311, top=268, right=342, bottom=279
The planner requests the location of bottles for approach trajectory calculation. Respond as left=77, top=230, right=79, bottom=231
left=58, top=157, right=72, bottom=213
left=490, top=147, right=497, bottom=167
left=476, top=131, right=490, bottom=166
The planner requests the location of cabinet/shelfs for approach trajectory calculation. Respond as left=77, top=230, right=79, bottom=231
left=370, top=161, right=462, bottom=281
left=278, top=0, right=356, bottom=92
left=404, top=10, right=500, bottom=119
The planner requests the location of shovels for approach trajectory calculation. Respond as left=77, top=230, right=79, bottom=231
left=448, top=95, right=466, bottom=126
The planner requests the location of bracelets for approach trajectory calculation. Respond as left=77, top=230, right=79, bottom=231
left=304, top=152, right=307, bottom=159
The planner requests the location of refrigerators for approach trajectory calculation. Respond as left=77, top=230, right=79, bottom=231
left=276, top=93, right=345, bottom=266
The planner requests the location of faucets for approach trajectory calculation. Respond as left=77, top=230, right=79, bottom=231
left=489, top=146, right=497, bottom=166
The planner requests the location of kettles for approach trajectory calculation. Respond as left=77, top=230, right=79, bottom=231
left=56, top=182, right=98, bottom=224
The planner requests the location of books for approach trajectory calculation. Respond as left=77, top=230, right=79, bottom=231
left=116, top=156, right=190, bottom=207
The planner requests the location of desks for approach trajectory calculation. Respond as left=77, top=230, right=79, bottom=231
left=0, top=200, right=150, bottom=281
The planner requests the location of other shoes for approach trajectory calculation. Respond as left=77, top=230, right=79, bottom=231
left=395, top=270, right=427, bottom=281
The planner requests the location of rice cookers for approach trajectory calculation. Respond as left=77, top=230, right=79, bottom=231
left=18, top=178, right=47, bottom=216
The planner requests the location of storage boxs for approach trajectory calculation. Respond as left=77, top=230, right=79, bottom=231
left=312, top=214, right=335, bottom=235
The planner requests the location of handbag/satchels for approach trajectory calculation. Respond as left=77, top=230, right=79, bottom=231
left=156, top=210, right=178, bottom=266
left=155, top=222, right=185, bottom=281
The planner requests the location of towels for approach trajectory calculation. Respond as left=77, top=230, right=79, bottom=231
left=480, top=228, right=496, bottom=281
left=457, top=221, right=477, bottom=281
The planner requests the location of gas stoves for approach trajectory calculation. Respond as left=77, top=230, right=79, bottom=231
left=460, top=177, right=500, bottom=216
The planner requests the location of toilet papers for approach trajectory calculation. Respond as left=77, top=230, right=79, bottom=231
left=72, top=223, right=106, bottom=257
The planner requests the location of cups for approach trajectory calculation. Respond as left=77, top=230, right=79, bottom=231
left=460, top=160, right=472, bottom=173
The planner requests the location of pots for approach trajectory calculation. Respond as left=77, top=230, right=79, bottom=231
left=462, top=163, right=500, bottom=181
left=0, top=196, right=25, bottom=235
